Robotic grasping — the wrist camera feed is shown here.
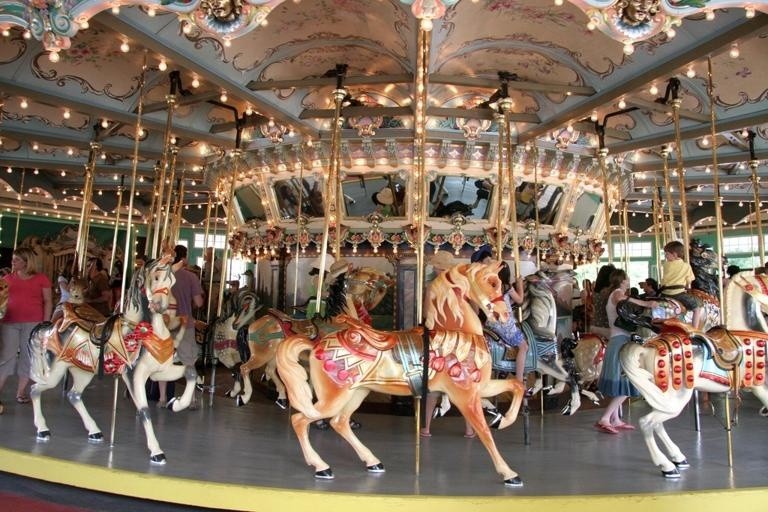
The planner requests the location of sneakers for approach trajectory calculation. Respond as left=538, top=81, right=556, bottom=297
left=189, top=402, right=199, bottom=410
left=157, top=400, right=167, bottom=408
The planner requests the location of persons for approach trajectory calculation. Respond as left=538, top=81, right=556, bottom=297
left=485, top=261, right=532, bottom=398
left=0, top=247, right=54, bottom=415
left=722, top=265, right=741, bottom=289
left=571, top=241, right=702, bottom=434
left=203, top=246, right=222, bottom=323
left=50, top=245, right=148, bottom=325
left=516, top=183, right=544, bottom=220
left=280, top=185, right=310, bottom=219
left=469, top=178, right=493, bottom=209
left=760, top=262, right=768, bottom=276
left=167, top=245, right=205, bottom=408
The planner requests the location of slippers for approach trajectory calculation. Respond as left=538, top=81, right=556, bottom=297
left=464, top=430, right=476, bottom=438
left=419, top=431, right=432, bottom=437
left=595, top=420, right=618, bottom=432
left=17, top=395, right=29, bottom=402
left=614, top=423, right=636, bottom=430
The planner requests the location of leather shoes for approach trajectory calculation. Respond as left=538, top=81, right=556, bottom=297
left=316, top=419, right=328, bottom=430
left=351, top=419, right=361, bottom=429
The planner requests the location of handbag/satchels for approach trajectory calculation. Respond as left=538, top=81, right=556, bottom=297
left=614, top=316, right=637, bottom=332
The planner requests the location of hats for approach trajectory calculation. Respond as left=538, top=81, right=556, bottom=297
left=431, top=251, right=453, bottom=270
left=326, top=260, right=348, bottom=284
left=471, top=245, right=491, bottom=263
